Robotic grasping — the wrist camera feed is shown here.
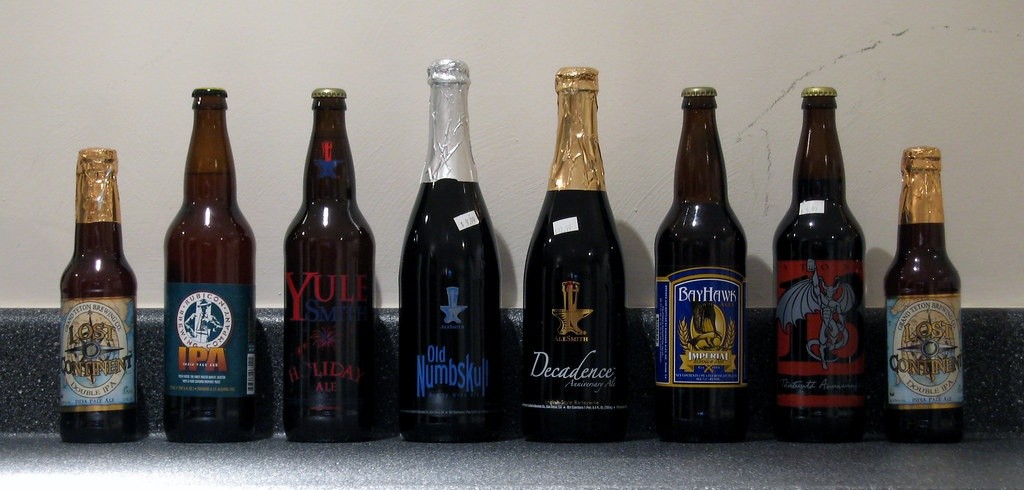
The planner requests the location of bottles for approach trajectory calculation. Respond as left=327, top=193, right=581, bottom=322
left=400, top=59, right=500, bottom=442
left=884, top=146, right=964, bottom=443
left=772, top=86, right=866, bottom=441
left=523, top=67, right=625, bottom=442
left=59, top=148, right=138, bottom=441
left=284, top=88, right=374, bottom=443
left=163, top=87, right=258, bottom=443
left=653, top=87, right=747, bottom=443
